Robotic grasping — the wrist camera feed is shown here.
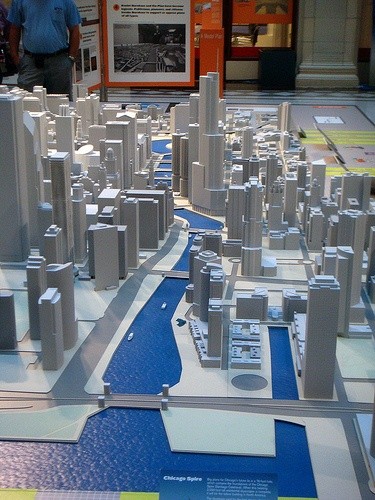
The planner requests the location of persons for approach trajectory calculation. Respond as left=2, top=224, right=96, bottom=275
left=6, top=0, right=82, bottom=102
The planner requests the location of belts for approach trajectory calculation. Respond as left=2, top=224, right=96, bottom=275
left=24, top=50, right=65, bottom=58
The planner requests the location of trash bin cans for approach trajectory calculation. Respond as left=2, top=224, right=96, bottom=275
left=258, top=47, right=297, bottom=90
